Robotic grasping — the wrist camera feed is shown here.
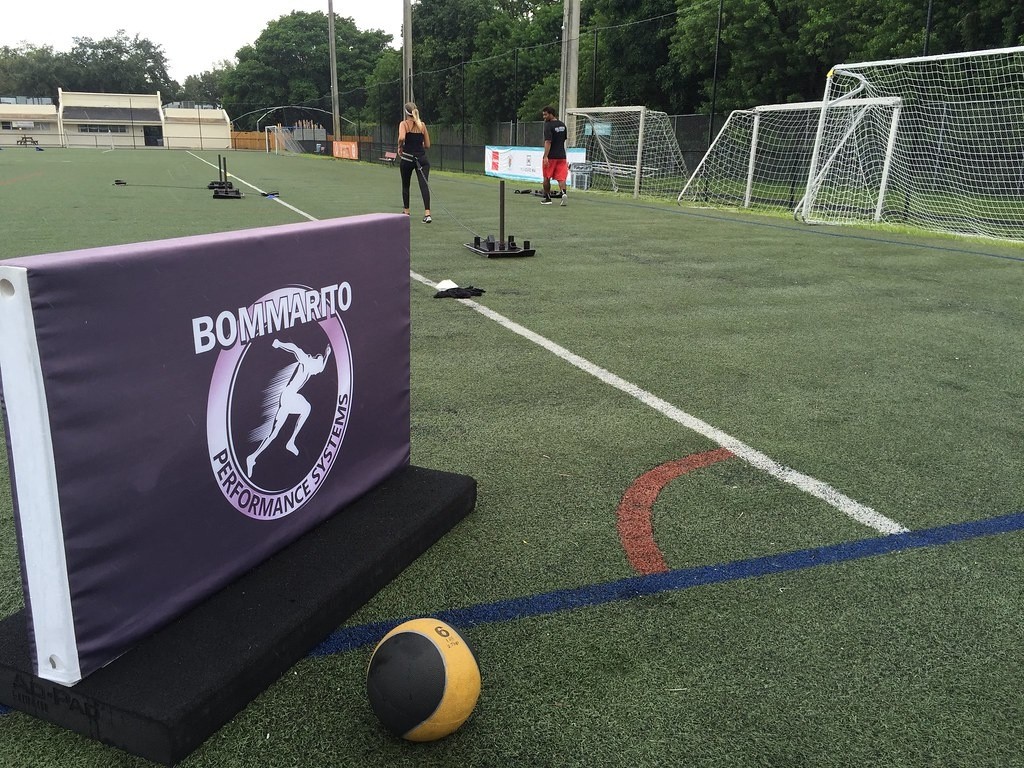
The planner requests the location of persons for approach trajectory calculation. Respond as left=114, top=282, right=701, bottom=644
left=398, top=102, right=433, bottom=224
left=540, top=107, right=569, bottom=206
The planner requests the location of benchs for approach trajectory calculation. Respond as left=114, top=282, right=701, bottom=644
left=16, top=140, right=38, bottom=145
left=379, top=152, right=397, bottom=168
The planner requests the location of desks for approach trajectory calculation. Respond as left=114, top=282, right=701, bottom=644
left=20, top=137, right=35, bottom=144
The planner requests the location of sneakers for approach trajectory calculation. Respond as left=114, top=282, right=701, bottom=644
left=540, top=198, right=552, bottom=204
left=402, top=212, right=411, bottom=216
left=560, top=194, right=568, bottom=205
left=421, top=215, right=432, bottom=224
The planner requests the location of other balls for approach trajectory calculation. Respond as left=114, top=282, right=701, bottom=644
left=366, top=617, right=482, bottom=742
